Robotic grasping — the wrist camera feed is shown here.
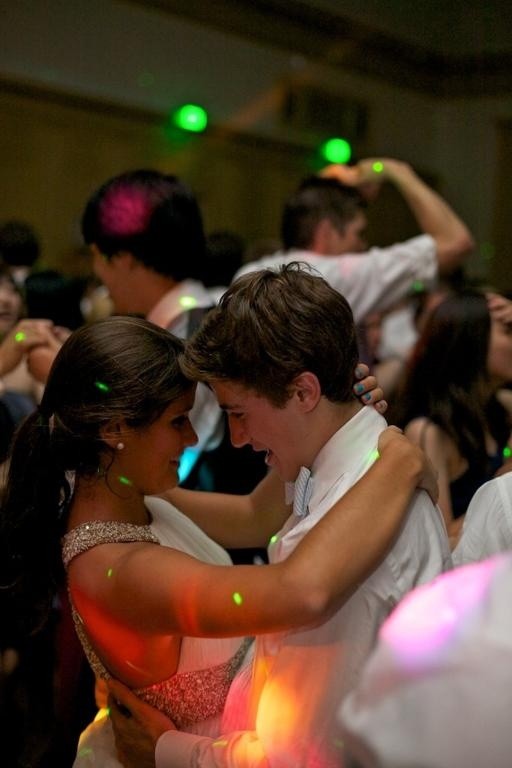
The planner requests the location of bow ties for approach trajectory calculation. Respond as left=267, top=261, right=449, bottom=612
left=281, top=465, right=318, bottom=520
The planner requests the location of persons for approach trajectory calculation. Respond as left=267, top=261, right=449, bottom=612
left=2, top=158, right=511, bottom=768
